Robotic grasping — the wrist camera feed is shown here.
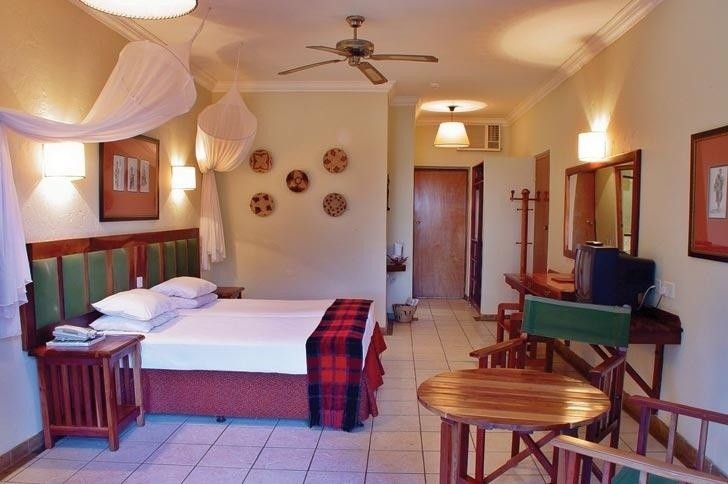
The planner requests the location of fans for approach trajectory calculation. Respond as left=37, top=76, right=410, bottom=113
left=278, top=15, right=438, bottom=85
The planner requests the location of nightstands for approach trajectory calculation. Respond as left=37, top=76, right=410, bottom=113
left=31, top=334, right=146, bottom=451
left=215, top=287, right=245, bottom=299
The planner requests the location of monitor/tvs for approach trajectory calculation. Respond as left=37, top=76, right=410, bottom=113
left=574, top=244, right=654, bottom=312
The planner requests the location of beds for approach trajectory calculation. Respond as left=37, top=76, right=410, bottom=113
left=20, top=228, right=388, bottom=431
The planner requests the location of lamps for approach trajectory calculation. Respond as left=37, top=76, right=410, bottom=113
left=578, top=132, right=606, bottom=160
left=42, top=142, right=86, bottom=181
left=171, top=166, right=196, bottom=190
left=79, top=0, right=199, bottom=18
left=434, top=105, right=469, bottom=147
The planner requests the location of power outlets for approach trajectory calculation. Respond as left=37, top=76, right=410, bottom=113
left=654, top=279, right=661, bottom=294
left=661, top=281, right=675, bottom=298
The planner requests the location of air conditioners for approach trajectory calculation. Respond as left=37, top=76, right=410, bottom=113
left=457, top=123, right=501, bottom=151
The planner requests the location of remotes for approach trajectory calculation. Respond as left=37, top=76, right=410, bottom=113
left=586, top=240, right=604, bottom=246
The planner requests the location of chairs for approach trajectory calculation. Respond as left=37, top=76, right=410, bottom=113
left=469, top=294, right=631, bottom=483
left=551, top=395, right=728, bottom=484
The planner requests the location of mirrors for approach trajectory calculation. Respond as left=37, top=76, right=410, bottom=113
left=562, top=149, right=641, bottom=258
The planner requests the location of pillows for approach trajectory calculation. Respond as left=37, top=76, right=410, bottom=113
left=89, top=312, right=178, bottom=333
left=91, top=289, right=176, bottom=321
left=171, top=293, right=218, bottom=309
left=150, top=276, right=218, bottom=298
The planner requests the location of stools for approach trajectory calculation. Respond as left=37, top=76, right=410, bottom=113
left=496, top=302, right=555, bottom=372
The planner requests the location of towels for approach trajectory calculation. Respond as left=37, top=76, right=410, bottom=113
left=395, top=243, right=403, bottom=259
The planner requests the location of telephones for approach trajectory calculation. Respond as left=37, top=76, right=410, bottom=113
left=52, top=324, right=97, bottom=341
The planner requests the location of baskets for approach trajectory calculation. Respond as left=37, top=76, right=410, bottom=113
left=392, top=303, right=416, bottom=323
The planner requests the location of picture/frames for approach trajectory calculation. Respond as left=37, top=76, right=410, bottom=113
left=99, top=135, right=160, bottom=222
left=688, top=126, right=728, bottom=263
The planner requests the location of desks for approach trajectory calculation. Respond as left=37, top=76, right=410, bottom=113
left=416, top=368, right=611, bottom=484
left=504, top=272, right=683, bottom=414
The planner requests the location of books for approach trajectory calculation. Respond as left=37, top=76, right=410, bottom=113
left=46, top=336, right=104, bottom=346
